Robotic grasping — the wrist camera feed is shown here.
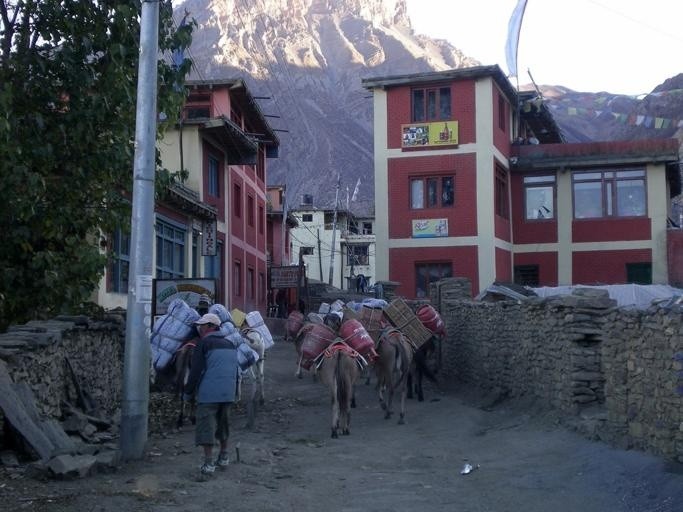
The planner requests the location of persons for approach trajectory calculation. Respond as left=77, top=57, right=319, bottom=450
left=182, top=314, right=238, bottom=474
left=284, top=302, right=297, bottom=340
left=356, top=274, right=367, bottom=295
left=299, top=296, right=306, bottom=314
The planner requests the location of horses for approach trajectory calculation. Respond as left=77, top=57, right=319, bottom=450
left=236, top=331, right=265, bottom=408
left=407, top=342, right=437, bottom=402
left=169, top=336, right=205, bottom=432
left=295, top=330, right=318, bottom=382
left=319, top=343, right=361, bottom=439
left=375, top=330, right=413, bottom=425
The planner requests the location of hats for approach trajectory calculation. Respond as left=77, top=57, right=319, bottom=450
left=193, top=314, right=221, bottom=326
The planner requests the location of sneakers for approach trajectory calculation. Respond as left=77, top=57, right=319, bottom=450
left=214, top=458, right=229, bottom=472
left=197, top=464, right=216, bottom=482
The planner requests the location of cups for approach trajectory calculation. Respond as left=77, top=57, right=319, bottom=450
left=439, top=131, right=444, bottom=140
left=435, top=219, right=446, bottom=233
left=449, top=128, right=453, bottom=139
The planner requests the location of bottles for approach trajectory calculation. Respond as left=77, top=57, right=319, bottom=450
left=443, top=122, right=449, bottom=140
